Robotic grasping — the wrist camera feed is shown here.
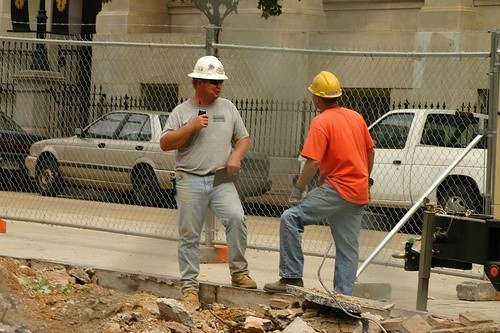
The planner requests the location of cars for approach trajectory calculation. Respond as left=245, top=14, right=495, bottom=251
left=0, top=112, right=50, bottom=192
left=24, top=110, right=273, bottom=210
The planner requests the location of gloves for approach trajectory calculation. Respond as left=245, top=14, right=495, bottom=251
left=288, top=185, right=307, bottom=206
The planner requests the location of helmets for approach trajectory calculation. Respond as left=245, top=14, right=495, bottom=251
left=187, top=56, right=228, bottom=80
left=307, top=70, right=342, bottom=98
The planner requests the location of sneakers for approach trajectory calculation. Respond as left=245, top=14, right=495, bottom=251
left=183, top=290, right=199, bottom=302
left=263, top=277, right=303, bottom=293
left=232, top=274, right=257, bottom=289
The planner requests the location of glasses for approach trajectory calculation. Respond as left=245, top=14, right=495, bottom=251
left=197, top=78, right=225, bottom=85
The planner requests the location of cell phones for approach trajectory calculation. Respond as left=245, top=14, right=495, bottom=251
left=199, top=110, right=206, bottom=116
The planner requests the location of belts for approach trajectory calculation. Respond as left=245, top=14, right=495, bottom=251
left=180, top=170, right=216, bottom=177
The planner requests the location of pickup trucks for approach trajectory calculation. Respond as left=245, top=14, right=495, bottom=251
left=295, top=108, right=487, bottom=217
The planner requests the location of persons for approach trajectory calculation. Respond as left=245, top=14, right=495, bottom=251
left=263, top=71, right=375, bottom=294
left=159, top=56, right=258, bottom=312
